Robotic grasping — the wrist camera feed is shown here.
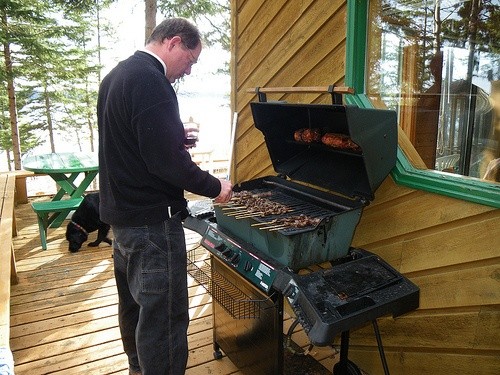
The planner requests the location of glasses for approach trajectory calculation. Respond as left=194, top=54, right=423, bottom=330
left=168, top=34, right=197, bottom=65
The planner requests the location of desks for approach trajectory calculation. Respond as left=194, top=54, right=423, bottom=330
left=23, top=152, right=99, bottom=228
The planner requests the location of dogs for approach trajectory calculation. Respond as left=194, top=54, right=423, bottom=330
left=65, top=192, right=112, bottom=253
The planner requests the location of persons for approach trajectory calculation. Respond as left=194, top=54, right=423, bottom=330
left=97, top=18, right=233, bottom=375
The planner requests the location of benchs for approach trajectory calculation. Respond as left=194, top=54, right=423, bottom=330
left=30, top=198, right=84, bottom=250
